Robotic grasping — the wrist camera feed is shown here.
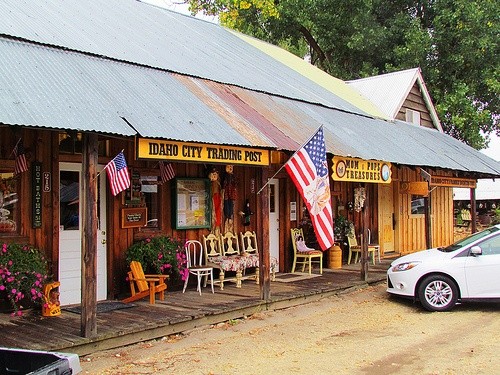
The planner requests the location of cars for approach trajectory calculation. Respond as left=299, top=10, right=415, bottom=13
left=385, top=223, right=500, bottom=313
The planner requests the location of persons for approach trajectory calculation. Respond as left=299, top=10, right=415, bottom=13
left=207, top=166, right=223, bottom=229
left=221, top=164, right=236, bottom=222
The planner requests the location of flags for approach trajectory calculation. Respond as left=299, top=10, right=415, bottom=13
left=285, top=127, right=334, bottom=252
left=13, top=140, right=28, bottom=175
left=157, top=159, right=175, bottom=184
left=105, top=149, right=131, bottom=196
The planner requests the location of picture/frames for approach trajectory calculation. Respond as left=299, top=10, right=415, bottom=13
left=121, top=206, right=148, bottom=229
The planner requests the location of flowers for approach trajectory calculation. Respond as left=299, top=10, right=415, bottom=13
left=113, top=234, right=190, bottom=281
left=0, top=241, right=51, bottom=317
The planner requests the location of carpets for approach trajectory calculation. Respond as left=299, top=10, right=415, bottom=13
left=59, top=300, right=138, bottom=314
left=270, top=272, right=322, bottom=283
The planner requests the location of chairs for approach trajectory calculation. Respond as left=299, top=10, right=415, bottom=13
left=183, top=231, right=279, bottom=296
left=345, top=222, right=381, bottom=265
left=290, top=227, right=323, bottom=275
left=121, top=260, right=170, bottom=305
